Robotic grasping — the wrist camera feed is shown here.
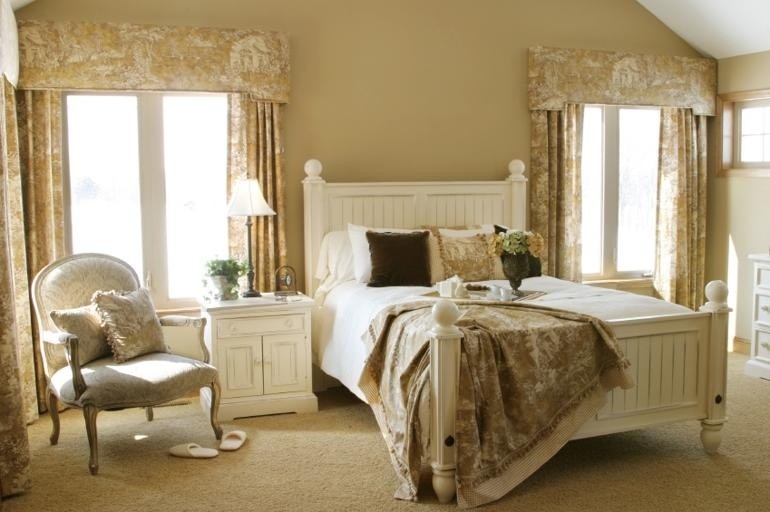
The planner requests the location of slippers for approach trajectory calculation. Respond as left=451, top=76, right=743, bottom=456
left=218, top=428, right=248, bottom=453
left=168, top=441, right=220, bottom=460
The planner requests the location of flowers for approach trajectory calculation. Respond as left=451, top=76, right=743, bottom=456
left=492, top=228, right=545, bottom=257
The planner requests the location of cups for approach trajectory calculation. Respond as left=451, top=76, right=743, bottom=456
left=491, top=283, right=513, bottom=301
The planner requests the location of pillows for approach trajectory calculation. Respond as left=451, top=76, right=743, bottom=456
left=93, top=287, right=171, bottom=363
left=49, top=303, right=112, bottom=367
left=318, top=224, right=540, bottom=287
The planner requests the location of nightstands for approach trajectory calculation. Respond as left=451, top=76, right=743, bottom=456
left=197, top=291, right=318, bottom=424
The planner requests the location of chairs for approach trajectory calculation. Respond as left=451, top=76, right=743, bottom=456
left=31, top=252, right=223, bottom=476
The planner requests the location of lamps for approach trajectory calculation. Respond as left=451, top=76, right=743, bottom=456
left=221, top=179, right=277, bottom=297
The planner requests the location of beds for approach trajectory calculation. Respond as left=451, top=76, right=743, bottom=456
left=301, top=159, right=733, bottom=508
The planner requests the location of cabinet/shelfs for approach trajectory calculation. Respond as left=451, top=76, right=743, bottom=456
left=747, top=254, right=769, bottom=381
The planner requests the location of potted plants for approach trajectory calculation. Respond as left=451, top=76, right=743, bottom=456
left=201, top=258, right=249, bottom=300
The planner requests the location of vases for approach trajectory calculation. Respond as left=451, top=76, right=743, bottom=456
left=500, top=251, right=529, bottom=295
